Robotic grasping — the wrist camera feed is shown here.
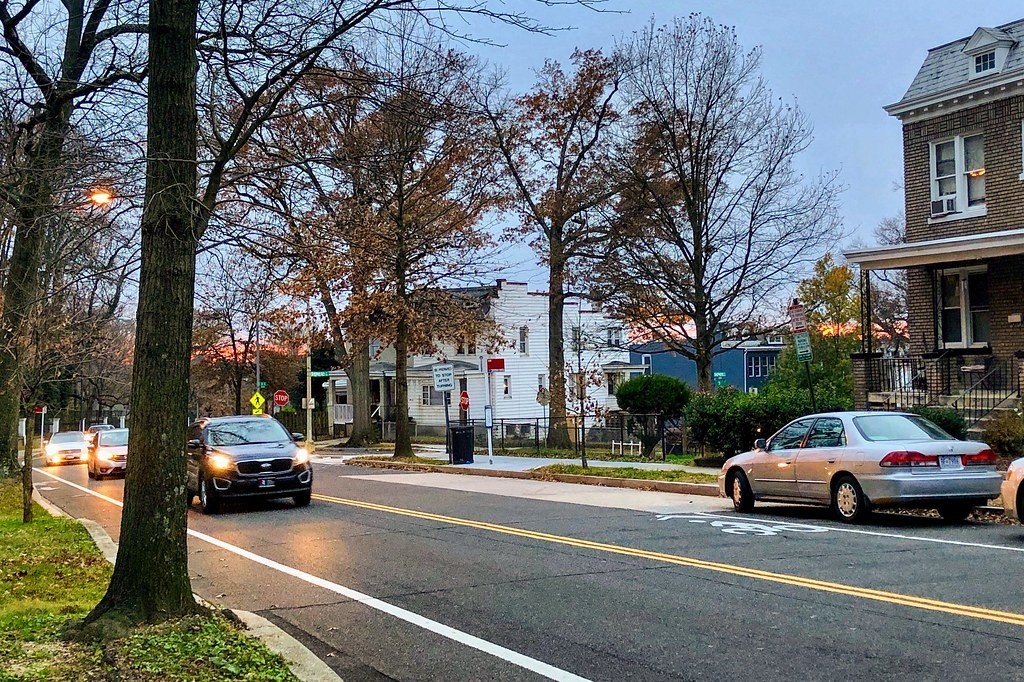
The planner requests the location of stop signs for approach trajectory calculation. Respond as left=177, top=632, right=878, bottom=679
left=274, top=390, right=289, bottom=406
left=460, top=392, right=469, bottom=411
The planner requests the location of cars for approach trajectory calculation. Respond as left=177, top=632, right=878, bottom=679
left=718, top=411, right=1003, bottom=526
left=1000, top=457, right=1024, bottom=526
left=189, top=414, right=314, bottom=514
left=87, top=428, right=129, bottom=481
left=45, top=433, right=90, bottom=465
left=85, top=424, right=115, bottom=449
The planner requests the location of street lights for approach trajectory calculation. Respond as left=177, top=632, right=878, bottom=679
left=24, top=190, right=113, bottom=523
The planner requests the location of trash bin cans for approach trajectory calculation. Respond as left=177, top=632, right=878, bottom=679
left=449, top=426, right=474, bottom=465
